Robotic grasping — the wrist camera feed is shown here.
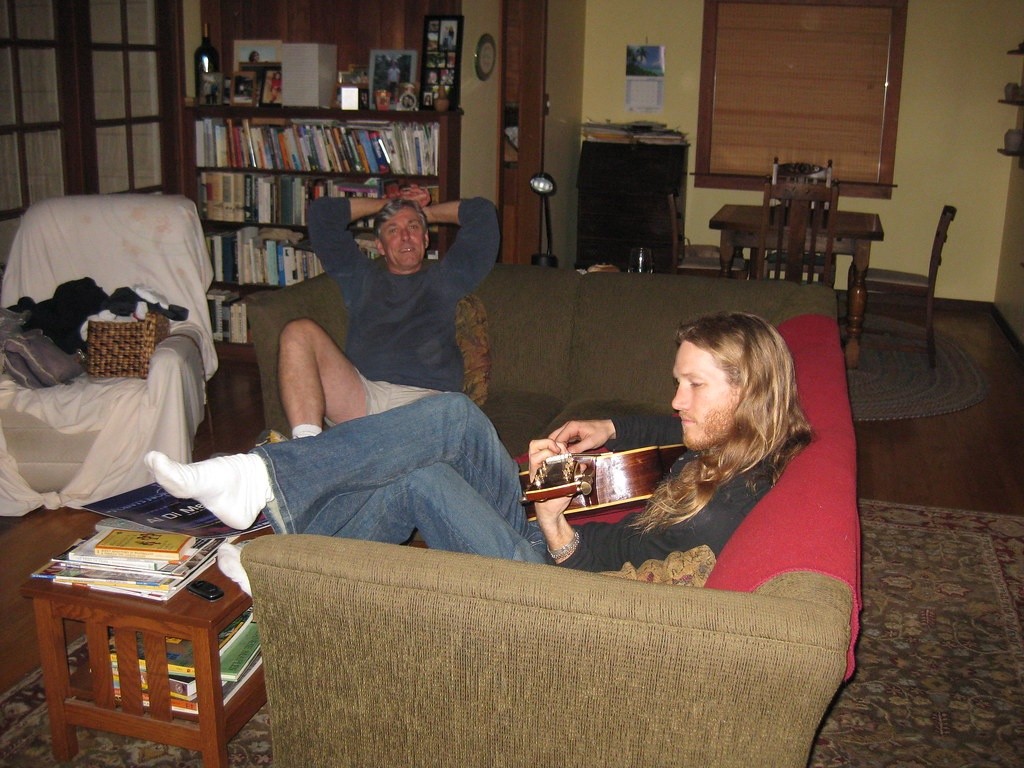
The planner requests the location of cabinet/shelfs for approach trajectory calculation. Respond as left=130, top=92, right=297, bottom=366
left=996, top=49, right=1024, bottom=158
left=183, top=106, right=463, bottom=363
left=575, top=141, right=689, bottom=274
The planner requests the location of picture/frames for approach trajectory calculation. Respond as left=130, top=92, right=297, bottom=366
left=368, top=49, right=419, bottom=111
left=228, top=39, right=283, bottom=107
left=419, top=14, right=465, bottom=109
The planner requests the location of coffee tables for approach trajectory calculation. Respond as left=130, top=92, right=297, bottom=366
left=19, top=525, right=277, bottom=768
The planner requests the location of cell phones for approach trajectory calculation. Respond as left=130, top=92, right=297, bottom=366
left=187, top=580, right=224, bottom=603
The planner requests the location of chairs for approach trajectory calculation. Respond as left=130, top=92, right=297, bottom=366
left=756, top=156, right=840, bottom=282
left=847, top=203, right=958, bottom=370
left=669, top=194, right=749, bottom=280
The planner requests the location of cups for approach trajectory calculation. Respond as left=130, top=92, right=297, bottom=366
left=375, top=90, right=391, bottom=111
left=628, top=247, right=653, bottom=275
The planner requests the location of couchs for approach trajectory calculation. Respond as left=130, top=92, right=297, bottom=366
left=240, top=257, right=860, bottom=767
left=0, top=192, right=219, bottom=517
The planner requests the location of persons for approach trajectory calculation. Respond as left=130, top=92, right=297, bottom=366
left=142, top=311, right=816, bottom=571
left=387, top=57, right=400, bottom=104
left=443, top=25, right=454, bottom=50
left=267, top=71, right=281, bottom=103
left=204, top=79, right=218, bottom=104
left=249, top=51, right=260, bottom=63
left=275, top=196, right=500, bottom=440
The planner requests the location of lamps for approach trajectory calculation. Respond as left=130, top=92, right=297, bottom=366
left=528, top=172, right=560, bottom=270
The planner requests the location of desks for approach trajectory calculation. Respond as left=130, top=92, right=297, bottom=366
left=708, top=204, right=886, bottom=370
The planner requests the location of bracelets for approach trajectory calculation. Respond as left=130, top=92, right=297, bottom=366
left=546, top=531, right=580, bottom=560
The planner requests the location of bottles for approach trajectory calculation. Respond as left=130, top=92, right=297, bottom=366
left=1004, top=128, right=1023, bottom=153
left=1004, top=83, right=1019, bottom=103
left=194, top=24, right=222, bottom=106
left=435, top=86, right=449, bottom=112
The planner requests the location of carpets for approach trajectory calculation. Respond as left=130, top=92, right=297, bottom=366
left=1, top=498, right=1023, bottom=768
left=834, top=308, right=984, bottom=423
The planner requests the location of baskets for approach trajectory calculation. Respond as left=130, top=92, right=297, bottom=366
left=86, top=312, right=171, bottom=379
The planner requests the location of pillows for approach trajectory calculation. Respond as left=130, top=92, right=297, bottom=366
left=452, top=294, right=488, bottom=408
left=597, top=544, right=717, bottom=590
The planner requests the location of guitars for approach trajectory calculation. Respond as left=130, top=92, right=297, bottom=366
left=518, top=444, right=688, bottom=523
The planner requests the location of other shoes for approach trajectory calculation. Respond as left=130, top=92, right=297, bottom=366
left=255, top=428, right=289, bottom=447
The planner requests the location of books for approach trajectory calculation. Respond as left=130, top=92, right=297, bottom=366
left=195, top=168, right=442, bottom=233
left=94, top=604, right=262, bottom=715
left=203, top=286, right=257, bottom=345
left=582, top=121, right=682, bottom=144
left=31, top=528, right=223, bottom=602
left=204, top=225, right=440, bottom=287
left=194, top=115, right=440, bottom=176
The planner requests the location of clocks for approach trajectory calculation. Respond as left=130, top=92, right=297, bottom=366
left=475, top=33, right=496, bottom=80
